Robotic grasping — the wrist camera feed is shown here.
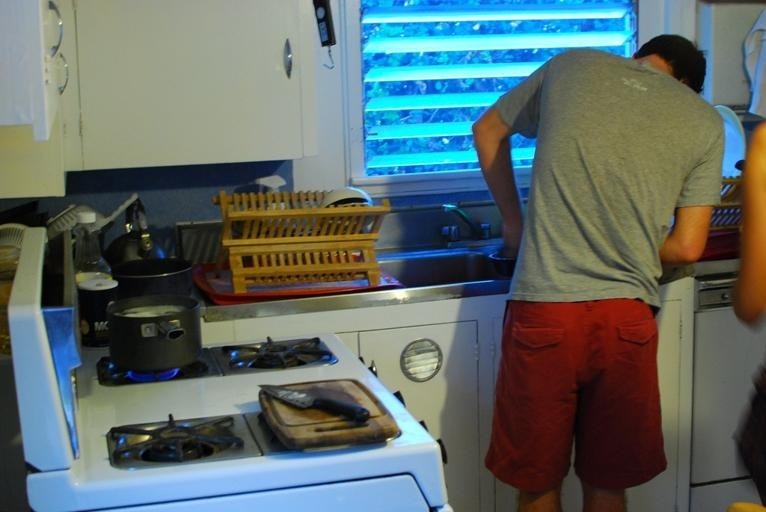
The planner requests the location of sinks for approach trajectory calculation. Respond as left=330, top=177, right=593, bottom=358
left=374, top=241, right=513, bottom=291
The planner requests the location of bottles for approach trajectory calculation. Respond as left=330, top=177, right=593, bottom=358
left=68, top=208, right=115, bottom=350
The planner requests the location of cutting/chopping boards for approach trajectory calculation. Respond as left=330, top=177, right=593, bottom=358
left=255, top=378, right=402, bottom=450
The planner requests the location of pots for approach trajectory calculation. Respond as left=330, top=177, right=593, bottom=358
left=100, top=296, right=205, bottom=371
left=110, top=259, right=194, bottom=295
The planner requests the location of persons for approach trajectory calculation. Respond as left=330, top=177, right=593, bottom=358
left=729, top=117, right=766, bottom=510
left=470, top=30, right=727, bottom=511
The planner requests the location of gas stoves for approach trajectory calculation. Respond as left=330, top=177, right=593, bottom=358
left=76, top=333, right=455, bottom=512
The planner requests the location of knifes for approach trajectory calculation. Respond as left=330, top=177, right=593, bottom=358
left=256, top=384, right=369, bottom=426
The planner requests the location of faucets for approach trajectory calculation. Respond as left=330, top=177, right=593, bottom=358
left=442, top=202, right=487, bottom=241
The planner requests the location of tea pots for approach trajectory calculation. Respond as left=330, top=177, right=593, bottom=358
left=109, top=196, right=182, bottom=267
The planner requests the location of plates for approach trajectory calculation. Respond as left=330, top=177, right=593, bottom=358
left=712, top=104, right=747, bottom=203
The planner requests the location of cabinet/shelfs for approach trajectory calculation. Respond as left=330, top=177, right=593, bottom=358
left=0, top=1, right=71, bottom=142
left=482, top=275, right=691, bottom=512
left=235, top=296, right=484, bottom=510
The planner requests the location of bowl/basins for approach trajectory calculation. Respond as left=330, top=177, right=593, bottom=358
left=316, top=185, right=374, bottom=224
